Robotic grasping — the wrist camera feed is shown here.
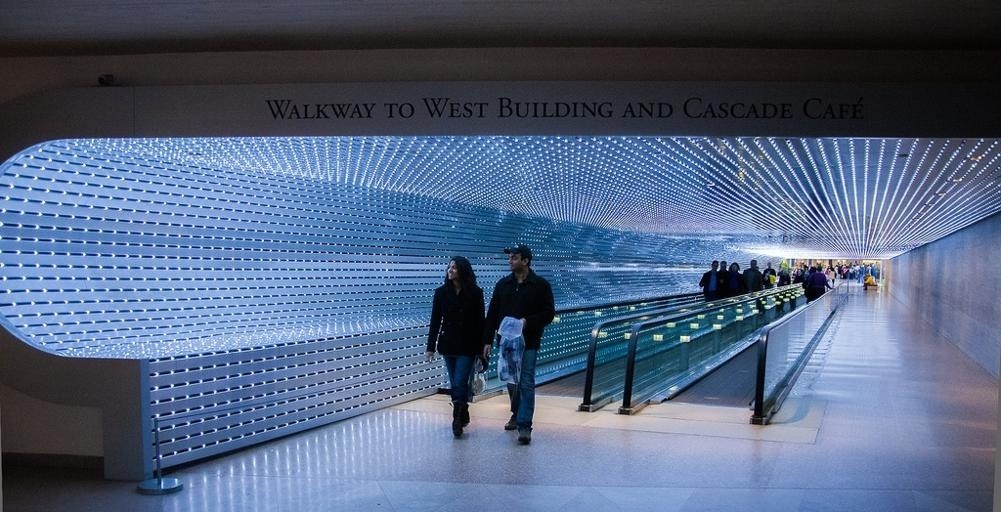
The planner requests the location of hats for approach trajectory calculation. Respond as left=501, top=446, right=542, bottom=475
left=503, top=243, right=534, bottom=261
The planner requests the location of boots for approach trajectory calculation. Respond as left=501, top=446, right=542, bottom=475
left=452, top=403, right=471, bottom=436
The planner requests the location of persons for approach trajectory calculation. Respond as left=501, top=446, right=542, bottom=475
left=699, top=260, right=877, bottom=304
left=426, top=245, right=555, bottom=443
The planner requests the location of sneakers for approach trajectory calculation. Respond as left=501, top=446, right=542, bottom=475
left=517, top=425, right=533, bottom=445
left=505, top=413, right=519, bottom=430
